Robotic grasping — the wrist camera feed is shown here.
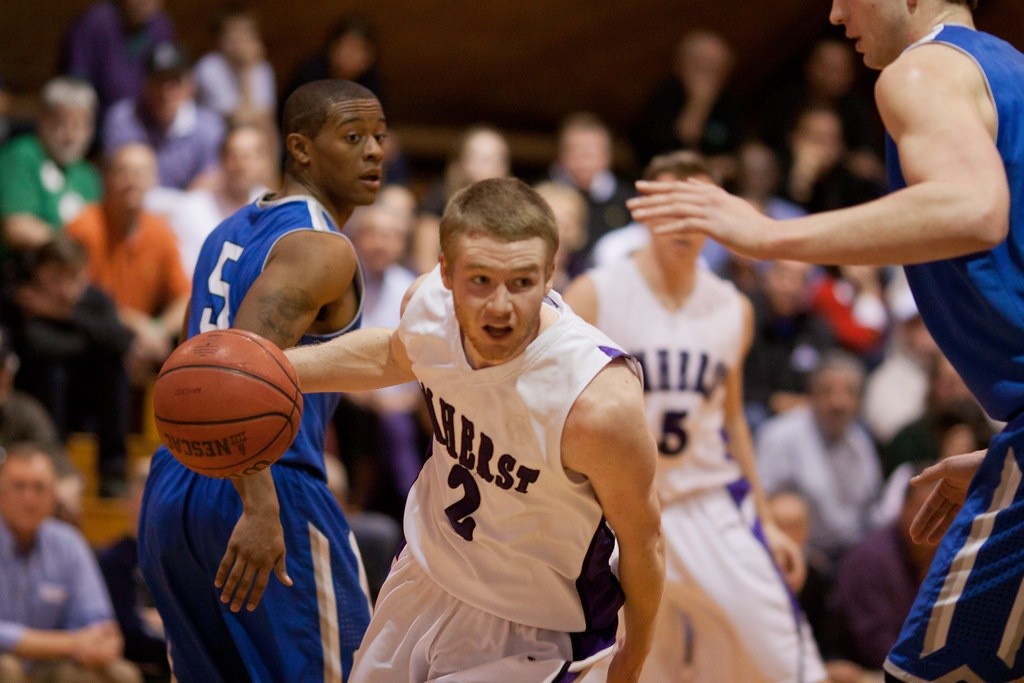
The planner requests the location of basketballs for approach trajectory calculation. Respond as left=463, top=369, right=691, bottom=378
left=154, top=329, right=303, bottom=478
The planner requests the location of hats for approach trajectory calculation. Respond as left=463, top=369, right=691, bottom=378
left=137, top=39, right=184, bottom=82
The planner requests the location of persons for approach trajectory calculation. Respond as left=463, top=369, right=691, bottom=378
left=185, top=106, right=277, bottom=235
left=0, top=0, right=1024, bottom=683
left=560, top=151, right=830, bottom=683
left=272, top=177, right=665, bottom=683
left=752, top=347, right=884, bottom=597
left=158, top=12, right=279, bottom=193
left=25, top=137, right=191, bottom=436
left=330, top=187, right=428, bottom=513
left=138, top=79, right=391, bottom=682
left=632, top=26, right=779, bottom=210
